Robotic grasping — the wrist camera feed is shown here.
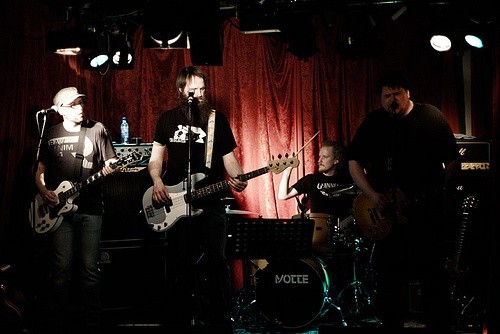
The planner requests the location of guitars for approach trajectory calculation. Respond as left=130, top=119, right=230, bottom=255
left=143, top=152, right=300, bottom=233
left=351, top=160, right=464, bottom=242
left=428, top=194, right=482, bottom=334
left=28, top=146, right=147, bottom=236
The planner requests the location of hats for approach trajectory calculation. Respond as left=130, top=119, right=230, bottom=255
left=53, top=87, right=86, bottom=109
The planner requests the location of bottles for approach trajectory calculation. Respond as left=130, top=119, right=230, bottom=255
left=120, top=117, right=130, bottom=144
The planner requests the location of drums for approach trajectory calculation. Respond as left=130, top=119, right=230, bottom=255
left=292, top=213, right=334, bottom=245
left=251, top=255, right=332, bottom=330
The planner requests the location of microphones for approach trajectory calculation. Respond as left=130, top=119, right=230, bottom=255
left=40, top=105, right=58, bottom=114
left=188, top=88, right=195, bottom=103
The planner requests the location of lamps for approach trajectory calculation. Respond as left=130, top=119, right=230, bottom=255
left=110, top=27, right=134, bottom=66
left=237, top=4, right=283, bottom=34
left=83, top=25, right=110, bottom=68
left=464, top=16, right=491, bottom=49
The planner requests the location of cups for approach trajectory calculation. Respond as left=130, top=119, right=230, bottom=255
left=131, top=137, right=142, bottom=144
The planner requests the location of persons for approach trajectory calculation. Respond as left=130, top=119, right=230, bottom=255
left=280, top=140, right=360, bottom=232
left=148, top=66, right=246, bottom=325
left=35, top=87, right=124, bottom=288
left=346, top=75, right=461, bottom=327
